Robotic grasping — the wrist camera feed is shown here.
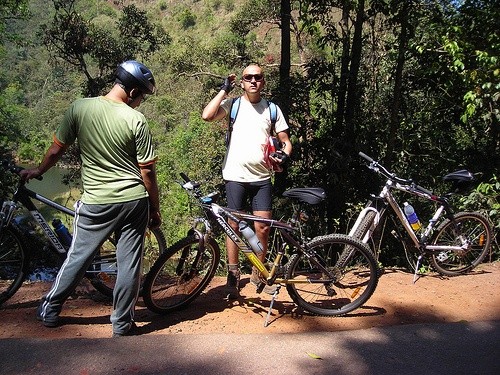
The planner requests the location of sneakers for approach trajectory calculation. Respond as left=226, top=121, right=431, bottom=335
left=250, top=267, right=279, bottom=294
left=226, top=270, right=240, bottom=299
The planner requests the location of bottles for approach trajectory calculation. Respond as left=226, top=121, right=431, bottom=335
left=403, top=201, right=421, bottom=230
left=52, top=218, right=72, bottom=247
left=239, top=221, right=263, bottom=254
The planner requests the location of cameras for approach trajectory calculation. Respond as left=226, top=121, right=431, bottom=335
left=270, top=150, right=289, bottom=161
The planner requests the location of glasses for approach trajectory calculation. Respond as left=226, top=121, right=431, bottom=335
left=138, top=94, right=147, bottom=103
left=242, top=75, right=264, bottom=82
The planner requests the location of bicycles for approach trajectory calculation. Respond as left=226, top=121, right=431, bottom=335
left=0, top=160, right=166, bottom=304
left=324, top=151, right=492, bottom=280
left=143, top=172, right=380, bottom=328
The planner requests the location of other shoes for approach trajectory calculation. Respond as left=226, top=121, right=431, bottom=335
left=36, top=307, right=58, bottom=327
left=112, top=323, right=145, bottom=335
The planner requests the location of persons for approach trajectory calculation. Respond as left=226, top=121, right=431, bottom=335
left=203, top=62, right=293, bottom=298
left=19, top=59, right=162, bottom=337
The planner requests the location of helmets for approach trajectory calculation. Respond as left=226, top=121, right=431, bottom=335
left=114, top=61, right=155, bottom=94
